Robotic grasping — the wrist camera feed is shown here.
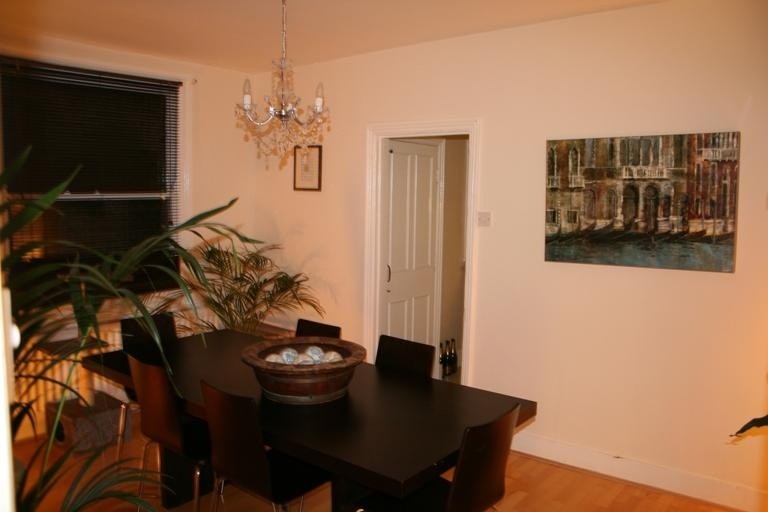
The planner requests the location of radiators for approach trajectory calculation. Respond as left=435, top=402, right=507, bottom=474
left=13, top=307, right=221, bottom=429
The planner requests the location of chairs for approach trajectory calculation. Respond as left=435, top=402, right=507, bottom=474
left=403, top=422, right=512, bottom=511
left=296, top=319, right=339, bottom=336
left=374, top=335, right=435, bottom=379
left=121, top=318, right=211, bottom=511
left=201, top=380, right=333, bottom=511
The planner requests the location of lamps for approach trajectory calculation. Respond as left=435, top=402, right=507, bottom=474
left=233, top=1, right=331, bottom=170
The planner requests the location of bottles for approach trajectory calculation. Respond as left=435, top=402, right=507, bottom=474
left=438, top=337, right=459, bottom=380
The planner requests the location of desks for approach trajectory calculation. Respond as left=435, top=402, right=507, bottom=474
left=81, top=316, right=538, bottom=511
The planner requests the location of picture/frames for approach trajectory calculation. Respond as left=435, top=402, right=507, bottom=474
left=293, top=145, right=322, bottom=191
